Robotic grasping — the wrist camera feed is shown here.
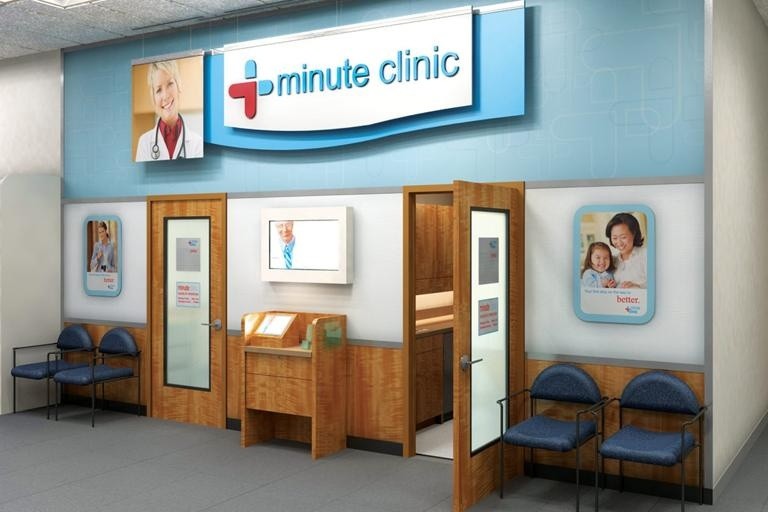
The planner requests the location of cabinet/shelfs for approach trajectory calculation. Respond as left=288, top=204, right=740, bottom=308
left=414, top=332, right=443, bottom=426
left=415, top=193, right=452, bottom=279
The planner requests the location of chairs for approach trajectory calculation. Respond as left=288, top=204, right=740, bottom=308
left=592, top=370, right=708, bottom=512
left=55, top=326, right=142, bottom=428
left=496, top=363, right=610, bottom=511
left=11, top=325, right=95, bottom=419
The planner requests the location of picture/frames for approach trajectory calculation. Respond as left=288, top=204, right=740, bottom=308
left=81, top=214, right=124, bottom=296
left=572, top=203, right=656, bottom=325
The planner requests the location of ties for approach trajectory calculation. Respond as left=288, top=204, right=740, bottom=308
left=284, top=246, right=291, bottom=269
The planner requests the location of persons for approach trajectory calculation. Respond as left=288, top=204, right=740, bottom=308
left=271, top=221, right=304, bottom=268
left=91, top=222, right=115, bottom=272
left=136, top=60, right=203, bottom=161
left=606, top=213, right=647, bottom=288
left=580, top=242, right=617, bottom=288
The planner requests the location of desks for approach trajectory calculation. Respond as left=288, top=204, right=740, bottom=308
left=240, top=309, right=347, bottom=459
